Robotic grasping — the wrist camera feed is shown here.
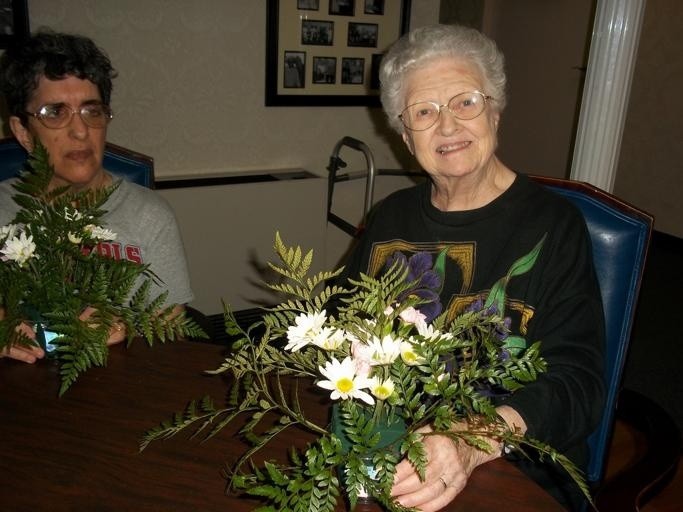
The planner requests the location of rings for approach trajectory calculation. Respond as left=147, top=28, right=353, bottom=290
left=112, top=320, right=125, bottom=332
left=439, top=477, right=448, bottom=488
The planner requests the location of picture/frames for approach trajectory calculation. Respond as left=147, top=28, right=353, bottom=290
left=264, top=0, right=411, bottom=107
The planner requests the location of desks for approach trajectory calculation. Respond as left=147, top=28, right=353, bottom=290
left=0, top=342, right=570, bottom=512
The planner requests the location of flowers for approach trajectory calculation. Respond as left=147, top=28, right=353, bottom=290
left=0, top=137, right=210, bottom=397
left=137, top=229, right=595, bottom=512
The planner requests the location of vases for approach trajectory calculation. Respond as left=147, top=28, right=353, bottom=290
left=329, top=404, right=404, bottom=488
left=39, top=322, right=64, bottom=357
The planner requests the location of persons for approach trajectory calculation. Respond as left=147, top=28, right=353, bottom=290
left=285, top=53, right=303, bottom=88
left=0, top=24, right=194, bottom=363
left=326, top=25, right=607, bottom=512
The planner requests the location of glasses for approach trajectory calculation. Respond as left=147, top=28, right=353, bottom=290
left=23, top=102, right=116, bottom=130
left=398, top=91, right=500, bottom=131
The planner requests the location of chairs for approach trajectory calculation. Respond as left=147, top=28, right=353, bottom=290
left=0, top=138, right=155, bottom=191
left=522, top=173, right=654, bottom=507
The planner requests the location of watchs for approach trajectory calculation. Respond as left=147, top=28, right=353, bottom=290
left=486, top=413, right=515, bottom=457
